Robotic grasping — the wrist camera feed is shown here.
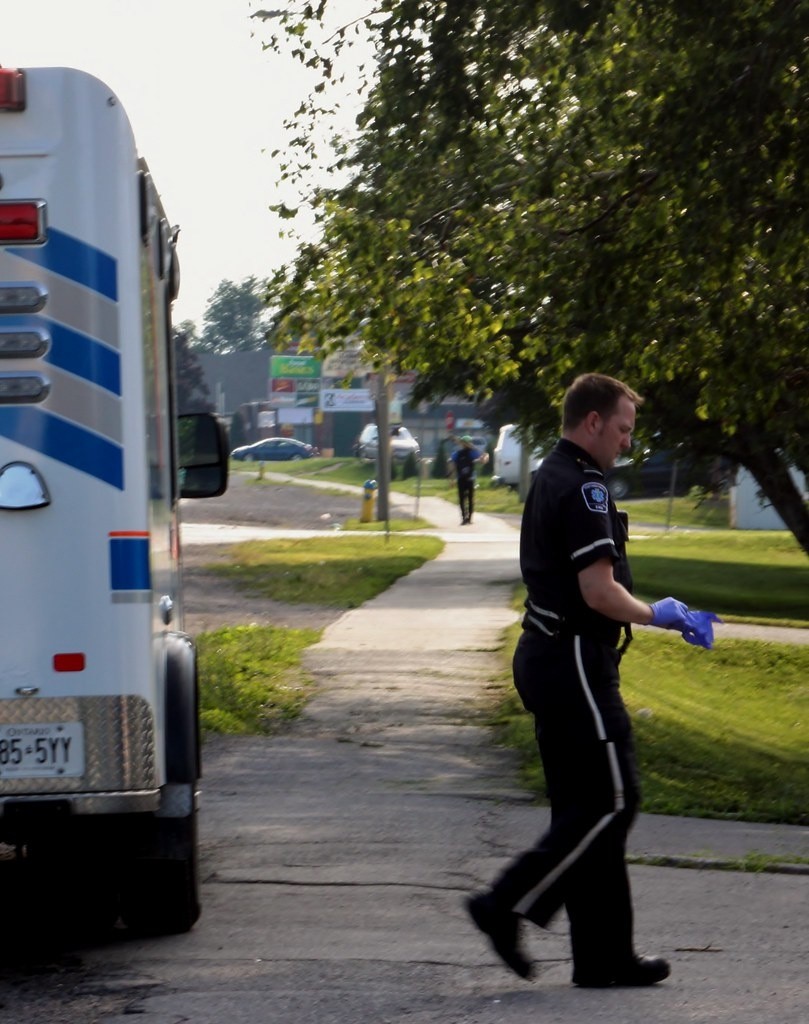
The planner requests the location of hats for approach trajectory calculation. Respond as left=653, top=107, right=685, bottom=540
left=460, top=436, right=472, bottom=443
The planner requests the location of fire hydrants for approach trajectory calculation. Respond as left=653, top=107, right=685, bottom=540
left=360, top=479, right=379, bottom=522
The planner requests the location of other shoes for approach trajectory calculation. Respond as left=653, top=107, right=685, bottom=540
left=464, top=890, right=536, bottom=982
left=574, top=952, right=672, bottom=990
left=461, top=517, right=469, bottom=525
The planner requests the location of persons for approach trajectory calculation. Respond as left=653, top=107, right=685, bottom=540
left=446, top=435, right=490, bottom=526
left=463, top=371, right=724, bottom=989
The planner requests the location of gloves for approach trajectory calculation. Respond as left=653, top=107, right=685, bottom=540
left=650, top=597, right=720, bottom=653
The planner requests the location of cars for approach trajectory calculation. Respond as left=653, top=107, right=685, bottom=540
left=234, top=435, right=319, bottom=464
left=604, top=441, right=734, bottom=501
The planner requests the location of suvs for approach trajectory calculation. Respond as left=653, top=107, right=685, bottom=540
left=494, top=423, right=551, bottom=490
left=355, top=423, right=421, bottom=463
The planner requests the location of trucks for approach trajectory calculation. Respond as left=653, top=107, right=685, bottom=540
left=0, top=64, right=233, bottom=932
